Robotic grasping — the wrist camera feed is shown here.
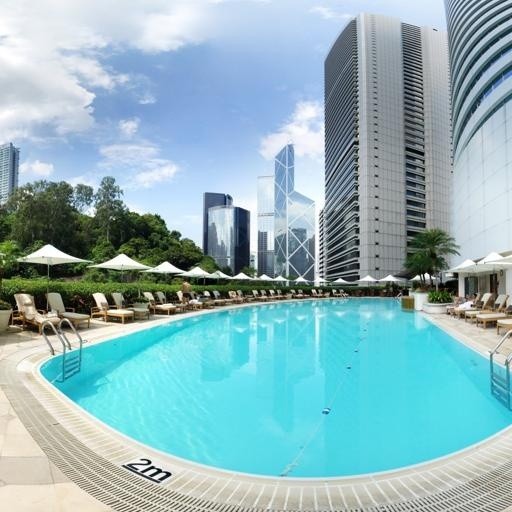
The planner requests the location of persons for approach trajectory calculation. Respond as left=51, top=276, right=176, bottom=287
left=181, top=280, right=192, bottom=307
left=468, top=292, right=480, bottom=303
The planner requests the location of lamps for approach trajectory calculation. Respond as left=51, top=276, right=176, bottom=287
left=499, top=269, right=503, bottom=277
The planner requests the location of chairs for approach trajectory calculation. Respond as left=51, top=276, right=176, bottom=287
left=14, top=293, right=61, bottom=334
left=447, top=290, right=512, bottom=338
left=143, top=292, right=176, bottom=315
left=47, top=293, right=91, bottom=330
left=91, top=292, right=134, bottom=325
left=156, top=291, right=187, bottom=314
left=110, top=292, right=150, bottom=320
left=175, top=289, right=348, bottom=310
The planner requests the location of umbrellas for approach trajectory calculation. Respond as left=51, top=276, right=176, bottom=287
left=205, top=270, right=436, bottom=297
left=15, top=243, right=92, bottom=311
left=462, top=252, right=511, bottom=308
left=442, top=259, right=493, bottom=295
left=174, top=266, right=214, bottom=299
left=484, top=255, right=511, bottom=266
left=140, top=260, right=186, bottom=304
left=86, top=253, right=150, bottom=307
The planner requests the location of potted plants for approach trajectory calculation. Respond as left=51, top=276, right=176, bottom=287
left=423, top=290, right=456, bottom=316
left=135, top=298, right=151, bottom=315
